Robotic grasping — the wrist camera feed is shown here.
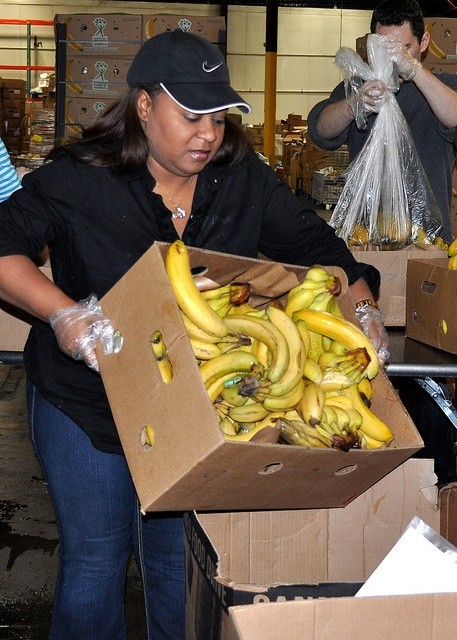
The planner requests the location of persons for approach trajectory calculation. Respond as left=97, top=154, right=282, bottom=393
left=306, top=1, right=456, bottom=246
left=0, top=139, right=50, bottom=266
left=0, top=29, right=390, bottom=639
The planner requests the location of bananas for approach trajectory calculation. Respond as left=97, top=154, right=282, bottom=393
left=140, top=239, right=395, bottom=452
left=346, top=215, right=457, bottom=268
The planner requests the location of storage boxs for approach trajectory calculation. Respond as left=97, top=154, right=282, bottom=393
left=66, top=57, right=135, bottom=99
left=423, top=17, right=457, bottom=63
left=423, top=64, right=457, bottom=74
left=78, top=240, right=428, bottom=515
left=56, top=13, right=142, bottom=57
left=277, top=113, right=348, bottom=194
left=142, top=14, right=226, bottom=47
left=247, top=124, right=264, bottom=153
left=185, top=460, right=457, bottom=640
left=65, top=99, right=115, bottom=138
left=227, top=592, right=457, bottom=640
left=351, top=246, right=449, bottom=327
left=406, top=258, right=457, bottom=353
left=0, top=78, right=31, bottom=169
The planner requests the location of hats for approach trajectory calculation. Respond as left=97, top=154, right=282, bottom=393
left=127, top=28, right=252, bottom=115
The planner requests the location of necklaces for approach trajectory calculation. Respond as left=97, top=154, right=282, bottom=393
left=156, top=176, right=193, bottom=219
